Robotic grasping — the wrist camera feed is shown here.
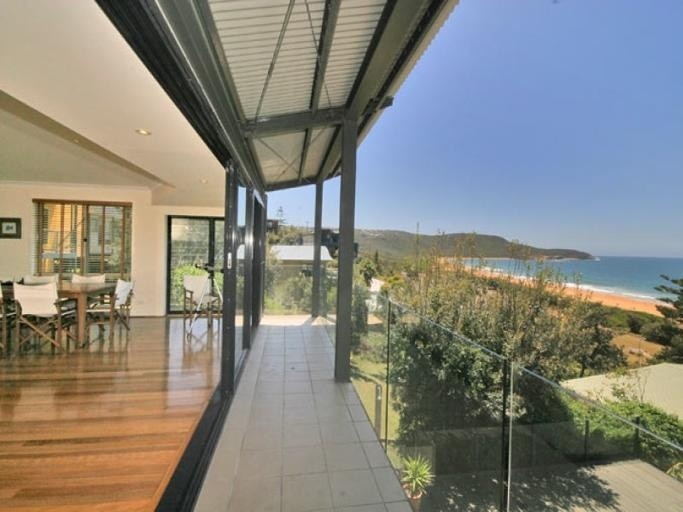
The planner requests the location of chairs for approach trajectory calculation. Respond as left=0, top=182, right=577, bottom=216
left=1, top=273, right=134, bottom=355
left=182, top=275, right=221, bottom=332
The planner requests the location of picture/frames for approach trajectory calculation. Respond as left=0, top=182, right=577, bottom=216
left=1, top=218, right=21, bottom=238
